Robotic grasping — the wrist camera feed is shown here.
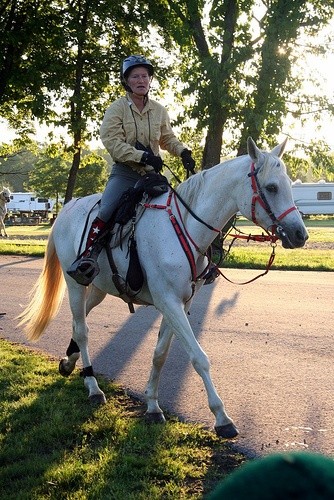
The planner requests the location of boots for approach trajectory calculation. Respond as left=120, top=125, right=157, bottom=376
left=74, top=216, right=111, bottom=286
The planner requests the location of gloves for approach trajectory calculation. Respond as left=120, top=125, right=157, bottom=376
left=181, top=148, right=195, bottom=170
left=141, top=151, right=163, bottom=172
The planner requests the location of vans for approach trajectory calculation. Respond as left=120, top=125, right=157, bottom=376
left=292, top=178, right=333, bottom=219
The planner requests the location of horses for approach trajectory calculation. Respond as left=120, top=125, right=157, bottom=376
left=12, top=133, right=311, bottom=439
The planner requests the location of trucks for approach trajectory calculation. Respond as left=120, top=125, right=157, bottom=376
left=6, top=192, right=52, bottom=216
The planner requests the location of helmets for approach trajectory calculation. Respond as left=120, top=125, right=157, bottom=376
left=121, top=55, right=154, bottom=91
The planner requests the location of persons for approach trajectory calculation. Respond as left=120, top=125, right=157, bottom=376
left=67, top=55, right=197, bottom=288
